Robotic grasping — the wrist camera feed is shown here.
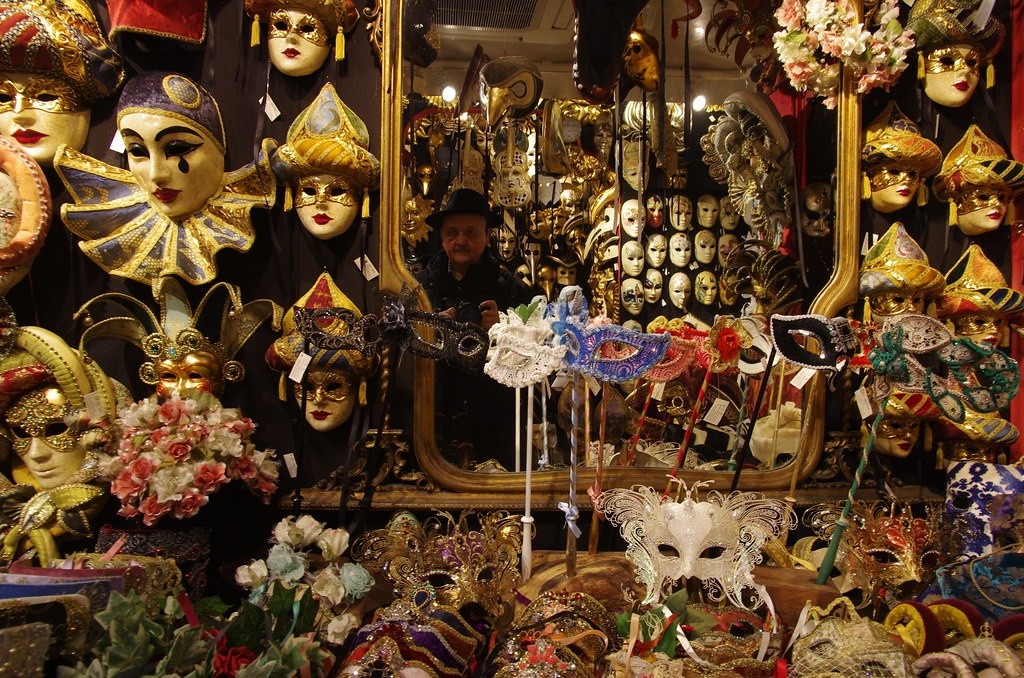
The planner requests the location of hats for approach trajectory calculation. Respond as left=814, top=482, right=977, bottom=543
left=424, top=189, right=505, bottom=229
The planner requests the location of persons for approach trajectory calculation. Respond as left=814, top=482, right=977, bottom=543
left=421, top=189, right=543, bottom=475
